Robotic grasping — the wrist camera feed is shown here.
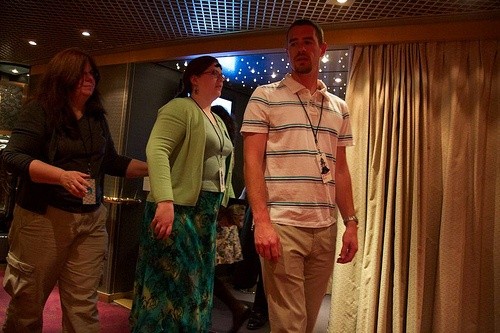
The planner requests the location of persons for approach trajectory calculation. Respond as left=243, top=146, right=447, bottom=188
left=132, top=55, right=255, bottom=333
left=209, top=104, right=270, bottom=333
left=236, top=18, right=359, bottom=333
left=0, top=48, right=154, bottom=333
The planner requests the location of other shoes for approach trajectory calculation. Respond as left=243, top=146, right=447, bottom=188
left=247, top=307, right=267, bottom=329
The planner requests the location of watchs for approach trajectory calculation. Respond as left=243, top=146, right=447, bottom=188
left=342, top=213, right=358, bottom=226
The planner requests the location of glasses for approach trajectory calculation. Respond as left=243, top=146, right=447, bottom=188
left=204, top=70, right=225, bottom=80
